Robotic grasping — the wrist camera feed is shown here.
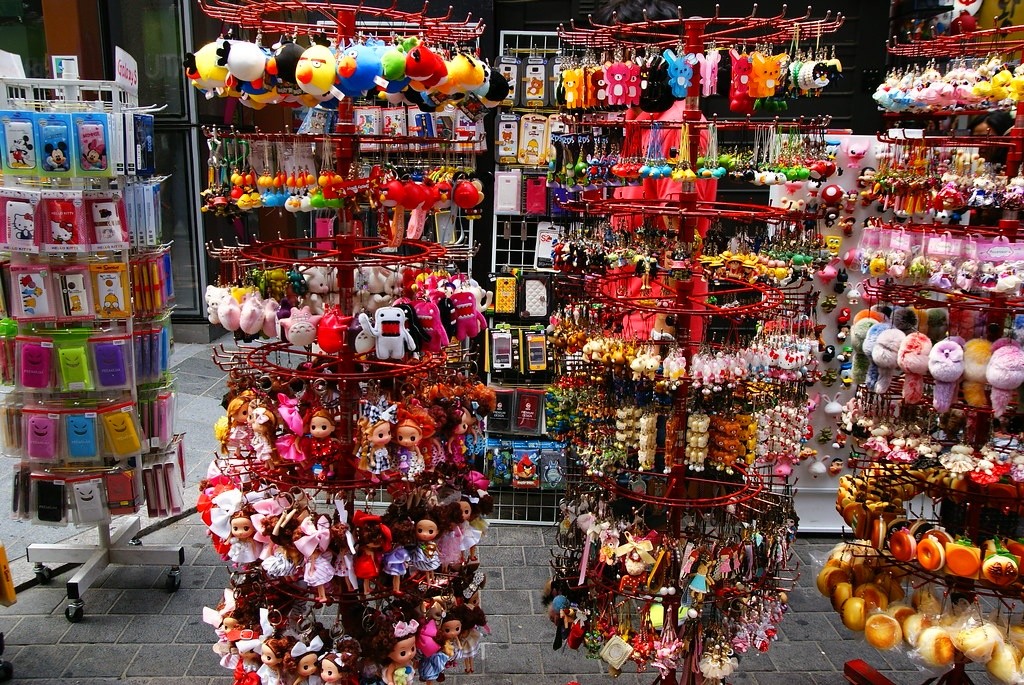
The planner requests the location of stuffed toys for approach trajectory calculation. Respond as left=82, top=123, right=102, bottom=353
left=184, top=32, right=1023, bottom=684
